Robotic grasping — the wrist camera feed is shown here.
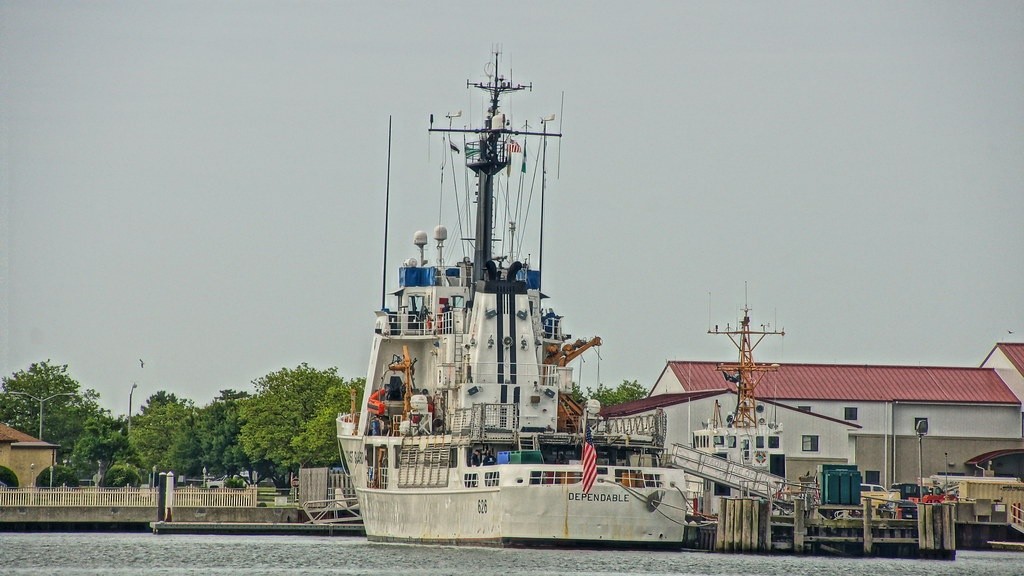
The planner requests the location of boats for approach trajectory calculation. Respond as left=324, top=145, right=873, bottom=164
left=334, top=42, right=687, bottom=550
left=686, top=279, right=794, bottom=518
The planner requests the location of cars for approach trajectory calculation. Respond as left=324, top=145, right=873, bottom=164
left=860, top=483, right=958, bottom=519
left=207, top=475, right=228, bottom=489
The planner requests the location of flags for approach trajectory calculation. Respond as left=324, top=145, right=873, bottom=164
left=451, top=145, right=459, bottom=154
left=583, top=419, right=598, bottom=495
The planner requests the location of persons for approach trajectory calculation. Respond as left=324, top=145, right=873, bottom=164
left=443, top=303, right=450, bottom=313
left=544, top=308, right=555, bottom=338
left=470, top=450, right=480, bottom=486
left=370, top=414, right=380, bottom=436
left=422, top=306, right=428, bottom=316
left=515, top=263, right=529, bottom=282
left=483, top=450, right=496, bottom=483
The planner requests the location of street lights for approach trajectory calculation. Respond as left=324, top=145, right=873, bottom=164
left=127, top=381, right=138, bottom=432
left=9, top=392, right=75, bottom=441
